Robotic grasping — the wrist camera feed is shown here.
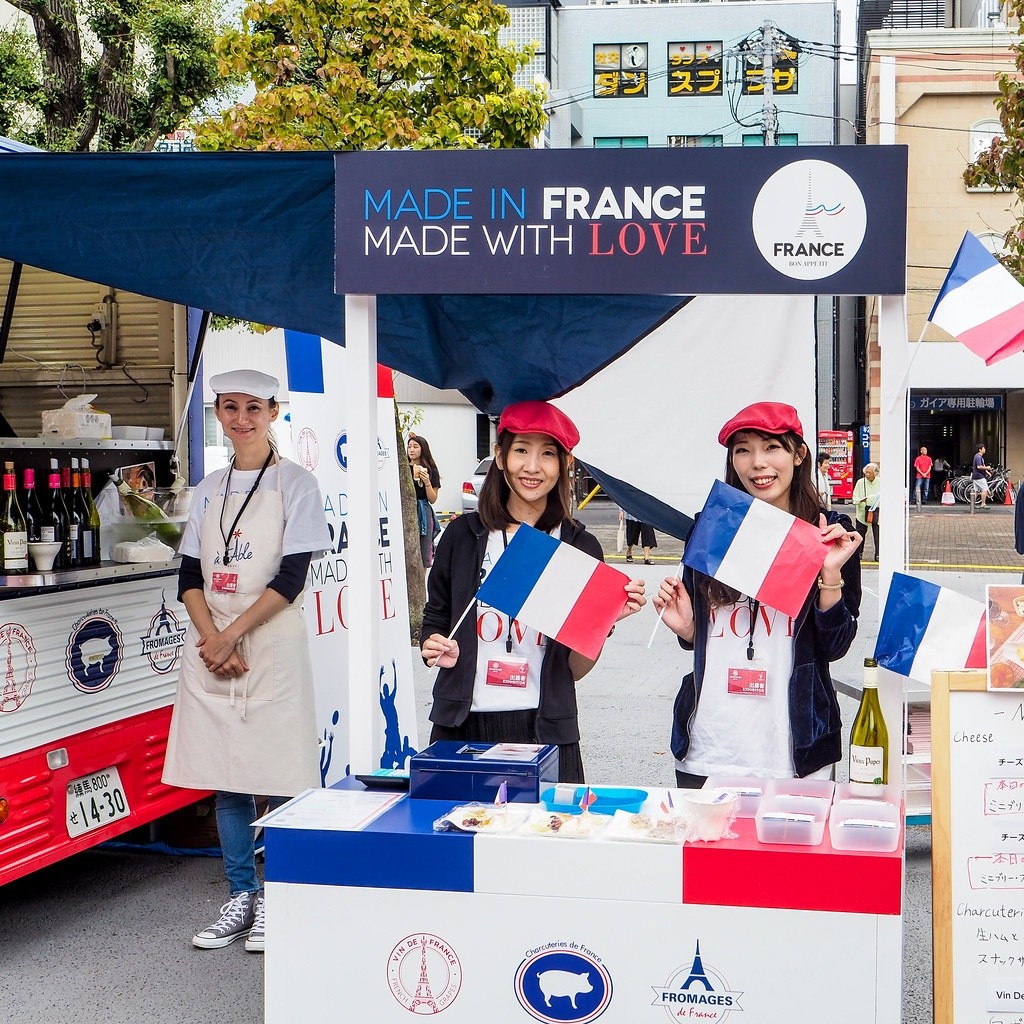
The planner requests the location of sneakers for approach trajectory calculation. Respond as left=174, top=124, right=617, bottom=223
left=192, top=891, right=255, bottom=949
left=244, top=890, right=265, bottom=951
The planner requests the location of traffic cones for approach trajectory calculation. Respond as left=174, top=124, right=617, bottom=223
left=1003, top=480, right=1014, bottom=506
left=940, top=480, right=955, bottom=506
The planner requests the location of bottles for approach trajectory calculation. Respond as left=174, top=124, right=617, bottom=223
left=82, top=458, right=100, bottom=568
left=820, top=438, right=847, bottom=462
left=847, top=658, right=889, bottom=785
left=0, top=461, right=28, bottom=576
left=155, top=476, right=186, bottom=514
left=106, top=468, right=182, bottom=546
left=21, top=468, right=45, bottom=571
left=40, top=458, right=70, bottom=572
left=69, top=458, right=92, bottom=569
left=61, top=467, right=73, bottom=521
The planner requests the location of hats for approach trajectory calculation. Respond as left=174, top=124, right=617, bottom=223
left=209, top=369, right=280, bottom=400
left=497, top=400, right=580, bottom=452
left=718, top=402, right=804, bottom=448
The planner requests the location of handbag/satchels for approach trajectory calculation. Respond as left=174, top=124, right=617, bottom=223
left=865, top=505, right=879, bottom=524
left=616, top=520, right=625, bottom=553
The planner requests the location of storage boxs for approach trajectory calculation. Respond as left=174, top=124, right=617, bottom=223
left=111, top=425, right=165, bottom=440
left=410, top=740, right=559, bottom=803
left=700, top=776, right=901, bottom=852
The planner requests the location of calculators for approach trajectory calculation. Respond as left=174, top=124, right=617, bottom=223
left=354, top=768, right=410, bottom=788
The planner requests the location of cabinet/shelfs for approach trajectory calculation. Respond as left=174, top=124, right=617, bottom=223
left=831, top=671, right=931, bottom=826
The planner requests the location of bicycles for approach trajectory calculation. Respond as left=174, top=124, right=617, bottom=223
left=940, top=460, right=1016, bottom=505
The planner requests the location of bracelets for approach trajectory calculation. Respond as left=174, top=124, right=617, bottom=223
left=818, top=575, right=845, bottom=590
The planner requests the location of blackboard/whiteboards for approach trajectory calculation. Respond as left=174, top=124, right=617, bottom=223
left=930, top=669, right=1024, bottom=1024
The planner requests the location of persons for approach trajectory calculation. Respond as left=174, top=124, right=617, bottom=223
left=814, top=450, right=832, bottom=512
left=420, top=399, right=647, bottom=791
left=971, top=443, right=993, bottom=510
left=176, top=369, right=330, bottom=953
left=406, top=434, right=439, bottom=584
left=851, top=463, right=880, bottom=562
left=621, top=504, right=658, bottom=566
left=913, top=447, right=933, bottom=506
left=653, top=400, right=865, bottom=794
left=933, top=455, right=951, bottom=498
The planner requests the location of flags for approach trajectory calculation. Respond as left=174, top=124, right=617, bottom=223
left=927, top=230, right=1024, bottom=366
left=681, top=480, right=839, bottom=618
left=475, top=523, right=635, bottom=661
left=869, top=569, right=989, bottom=687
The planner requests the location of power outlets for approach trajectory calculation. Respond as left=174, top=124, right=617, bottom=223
left=95, top=303, right=110, bottom=325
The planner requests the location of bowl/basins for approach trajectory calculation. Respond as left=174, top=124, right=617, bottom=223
left=110, top=519, right=189, bottom=559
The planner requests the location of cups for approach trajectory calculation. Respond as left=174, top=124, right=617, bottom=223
left=27, top=542, right=62, bottom=571
left=683, top=791, right=737, bottom=841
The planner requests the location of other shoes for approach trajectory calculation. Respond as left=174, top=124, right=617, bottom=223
left=874, top=555, right=879, bottom=562
left=643, top=558, right=655, bottom=565
left=975, top=505, right=981, bottom=509
left=981, top=505, right=991, bottom=509
left=922, top=500, right=928, bottom=504
left=626, top=555, right=633, bottom=562
left=910, top=499, right=916, bottom=504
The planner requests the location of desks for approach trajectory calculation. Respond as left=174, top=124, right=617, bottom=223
left=262, top=776, right=904, bottom=1024
